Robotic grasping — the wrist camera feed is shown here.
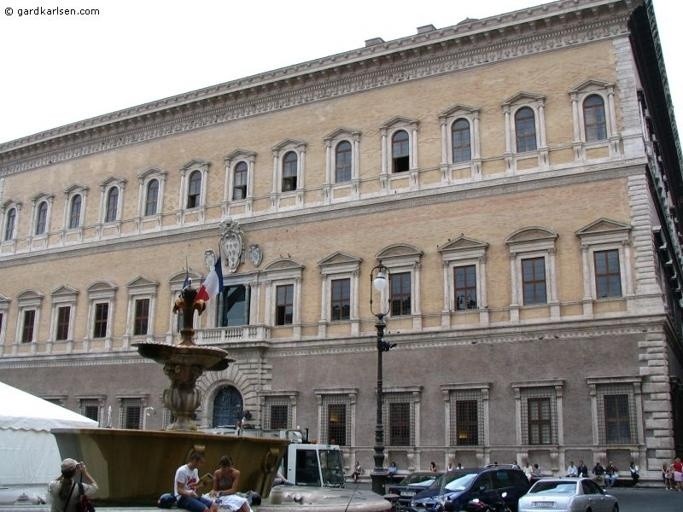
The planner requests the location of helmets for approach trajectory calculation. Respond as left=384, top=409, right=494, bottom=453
left=157, top=493, right=177, bottom=508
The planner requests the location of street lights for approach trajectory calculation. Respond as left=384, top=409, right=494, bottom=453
left=369, top=258, right=398, bottom=495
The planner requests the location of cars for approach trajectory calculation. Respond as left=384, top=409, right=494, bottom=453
left=383, top=471, right=442, bottom=511
left=516, top=471, right=621, bottom=512
left=409, top=462, right=530, bottom=512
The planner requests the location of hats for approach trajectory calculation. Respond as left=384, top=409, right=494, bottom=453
left=61, top=458, right=80, bottom=470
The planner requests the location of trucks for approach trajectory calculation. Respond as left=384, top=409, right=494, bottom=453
left=196, top=423, right=347, bottom=490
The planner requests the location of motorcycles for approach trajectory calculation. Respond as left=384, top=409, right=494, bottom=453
left=465, top=484, right=514, bottom=512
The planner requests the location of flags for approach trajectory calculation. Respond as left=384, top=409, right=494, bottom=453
left=193, top=255, right=225, bottom=304
left=182, top=265, right=191, bottom=290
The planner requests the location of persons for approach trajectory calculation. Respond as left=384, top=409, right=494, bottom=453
left=209, top=454, right=250, bottom=512
left=351, top=461, right=361, bottom=484
left=428, top=462, right=437, bottom=473
left=566, top=460, right=639, bottom=488
left=511, top=459, right=542, bottom=483
left=385, top=461, right=399, bottom=474
left=47, top=457, right=99, bottom=512
left=662, top=456, right=683, bottom=493
left=446, top=462, right=465, bottom=472
left=173, top=451, right=218, bottom=512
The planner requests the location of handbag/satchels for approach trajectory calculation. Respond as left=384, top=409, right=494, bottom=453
left=78, top=495, right=95, bottom=512
left=247, top=491, right=261, bottom=505
left=665, top=474, right=672, bottom=478
